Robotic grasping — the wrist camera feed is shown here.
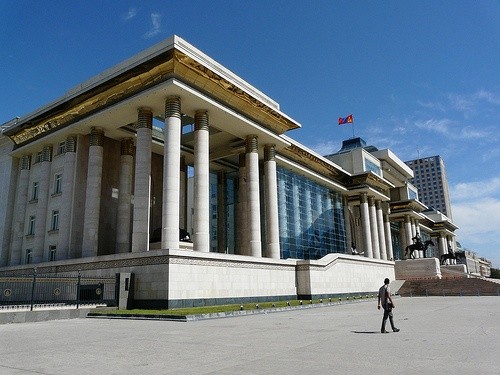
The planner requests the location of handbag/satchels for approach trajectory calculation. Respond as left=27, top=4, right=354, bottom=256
left=384, top=302, right=394, bottom=312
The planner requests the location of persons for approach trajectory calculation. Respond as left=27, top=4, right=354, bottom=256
left=448, top=245, right=455, bottom=258
left=411, top=233, right=424, bottom=251
left=378, top=278, right=400, bottom=333
left=390, top=258, right=392, bottom=261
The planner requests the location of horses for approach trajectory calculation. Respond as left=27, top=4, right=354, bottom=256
left=440, top=251, right=461, bottom=265
left=404, top=239, right=434, bottom=259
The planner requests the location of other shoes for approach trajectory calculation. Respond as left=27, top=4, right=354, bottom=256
left=382, top=330, right=391, bottom=334
left=392, top=328, right=401, bottom=333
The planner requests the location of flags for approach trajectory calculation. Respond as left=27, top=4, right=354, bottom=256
left=339, top=114, right=352, bottom=125
